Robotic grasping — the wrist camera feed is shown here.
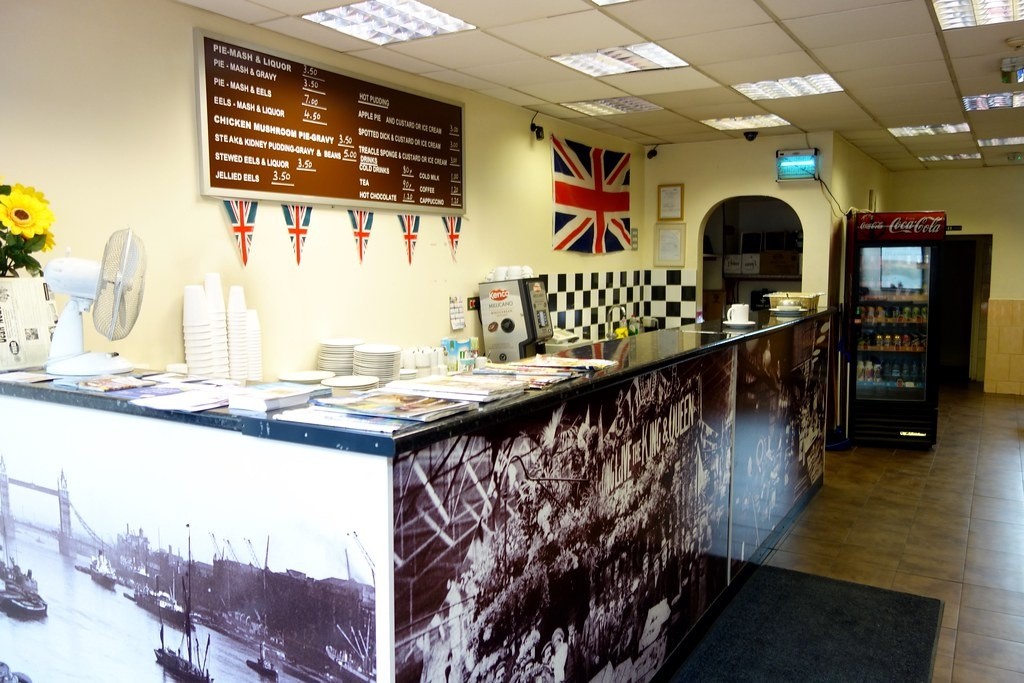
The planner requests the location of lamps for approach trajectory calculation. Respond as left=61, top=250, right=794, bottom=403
left=647, top=145, right=657, bottom=159
left=531, top=124, right=544, bottom=140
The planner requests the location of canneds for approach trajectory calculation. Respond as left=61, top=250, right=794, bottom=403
left=859, top=305, right=926, bottom=347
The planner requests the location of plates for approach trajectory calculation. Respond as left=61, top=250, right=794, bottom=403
left=722, top=320, right=756, bottom=329
left=485, top=265, right=534, bottom=281
left=768, top=308, right=808, bottom=314
left=277, top=338, right=418, bottom=391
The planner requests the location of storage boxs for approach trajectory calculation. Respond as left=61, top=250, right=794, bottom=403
left=724, top=251, right=798, bottom=274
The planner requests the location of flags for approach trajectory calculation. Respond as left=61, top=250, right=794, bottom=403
left=550, top=132, right=631, bottom=254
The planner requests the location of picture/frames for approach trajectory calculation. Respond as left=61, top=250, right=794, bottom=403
left=652, top=222, right=687, bottom=267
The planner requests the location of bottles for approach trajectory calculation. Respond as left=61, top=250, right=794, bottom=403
left=857, top=360, right=918, bottom=383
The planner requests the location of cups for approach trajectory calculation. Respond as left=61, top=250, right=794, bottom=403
left=726, top=303, right=749, bottom=323
left=475, top=356, right=492, bottom=369
left=182, top=272, right=263, bottom=382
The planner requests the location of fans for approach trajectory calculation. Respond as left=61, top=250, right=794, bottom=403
left=45, top=227, right=147, bottom=375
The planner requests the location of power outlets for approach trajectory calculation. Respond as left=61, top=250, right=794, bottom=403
left=630, top=228, right=639, bottom=252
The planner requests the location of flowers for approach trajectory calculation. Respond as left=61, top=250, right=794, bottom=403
left=0, top=176, right=55, bottom=278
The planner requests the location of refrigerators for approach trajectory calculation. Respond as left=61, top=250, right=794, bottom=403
left=840, top=210, right=947, bottom=450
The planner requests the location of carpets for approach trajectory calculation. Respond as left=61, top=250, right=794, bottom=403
left=649, top=565, right=946, bottom=683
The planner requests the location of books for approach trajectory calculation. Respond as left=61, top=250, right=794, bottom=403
left=0, top=354, right=619, bottom=433
